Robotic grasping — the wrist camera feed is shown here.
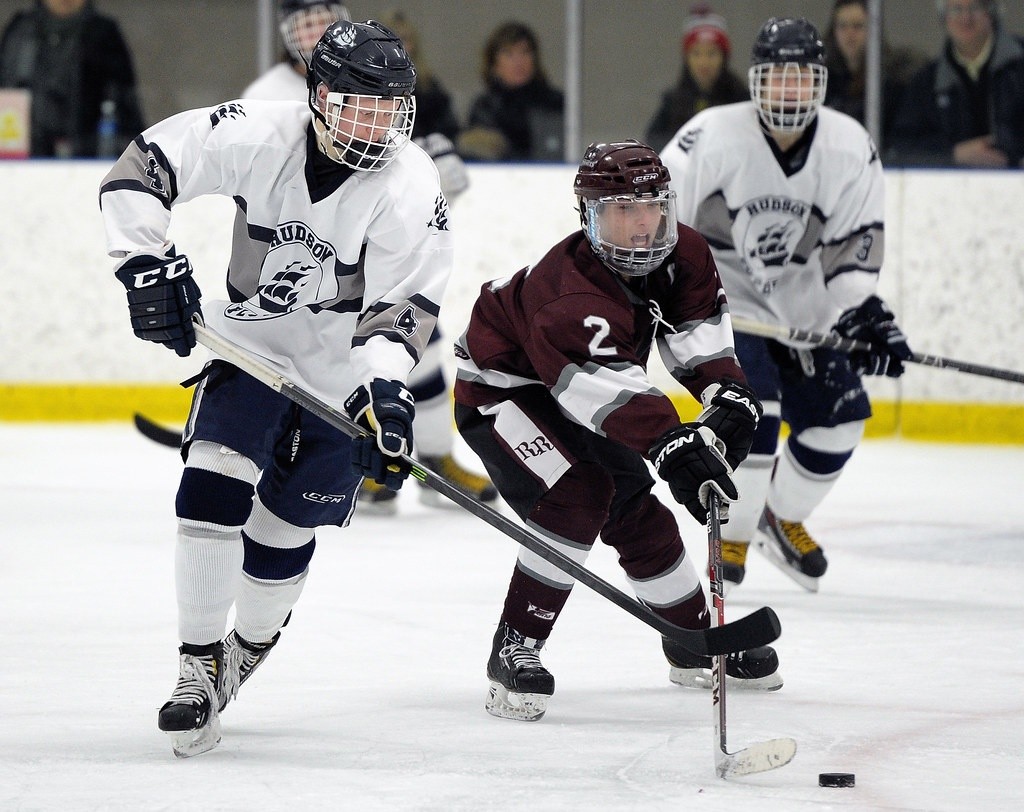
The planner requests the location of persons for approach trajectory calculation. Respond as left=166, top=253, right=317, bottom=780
left=1, top=0, right=1024, bottom=167
left=103, top=20, right=449, bottom=761
left=454, top=140, right=785, bottom=720
left=656, top=15, right=914, bottom=602
left=228, top=1, right=499, bottom=504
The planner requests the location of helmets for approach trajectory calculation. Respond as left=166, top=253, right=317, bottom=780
left=298, top=18, right=417, bottom=172
left=573, top=136, right=680, bottom=277
left=747, top=16, right=829, bottom=134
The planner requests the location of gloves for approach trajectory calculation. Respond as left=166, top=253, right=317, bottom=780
left=703, top=377, right=763, bottom=472
left=111, top=239, right=207, bottom=357
left=343, top=376, right=416, bottom=490
left=645, top=423, right=739, bottom=525
left=830, top=293, right=913, bottom=377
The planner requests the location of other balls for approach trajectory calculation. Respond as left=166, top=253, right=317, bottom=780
left=819, top=772, right=856, bottom=788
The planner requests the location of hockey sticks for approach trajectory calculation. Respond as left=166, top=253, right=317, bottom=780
left=708, top=490, right=796, bottom=777
left=191, top=318, right=783, bottom=656
left=731, top=319, right=1024, bottom=384
left=131, top=410, right=184, bottom=449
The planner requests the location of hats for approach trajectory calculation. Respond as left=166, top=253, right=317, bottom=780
left=681, top=4, right=729, bottom=53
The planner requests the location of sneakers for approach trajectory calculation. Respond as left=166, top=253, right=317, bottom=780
left=414, top=451, right=499, bottom=509
left=484, top=616, right=554, bottom=722
left=706, top=537, right=753, bottom=596
left=661, top=633, right=786, bottom=685
left=157, top=638, right=226, bottom=756
left=355, top=476, right=399, bottom=516
left=211, top=608, right=294, bottom=711
left=751, top=502, right=828, bottom=592
